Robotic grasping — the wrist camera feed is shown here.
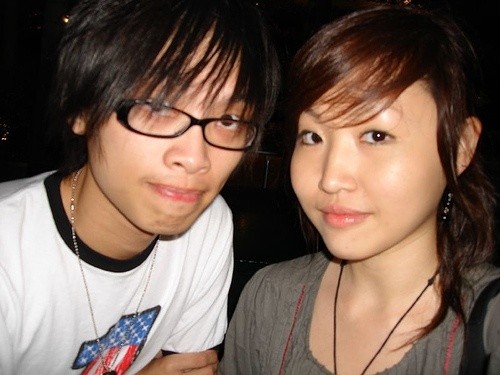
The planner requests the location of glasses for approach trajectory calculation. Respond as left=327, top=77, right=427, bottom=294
left=111, top=99, right=259, bottom=150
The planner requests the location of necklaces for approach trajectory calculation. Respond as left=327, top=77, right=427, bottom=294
left=332, top=261, right=444, bottom=373
left=72, top=169, right=159, bottom=374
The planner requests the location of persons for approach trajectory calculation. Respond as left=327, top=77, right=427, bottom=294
left=217, top=0, right=500, bottom=375
left=1, top=1, right=281, bottom=375
